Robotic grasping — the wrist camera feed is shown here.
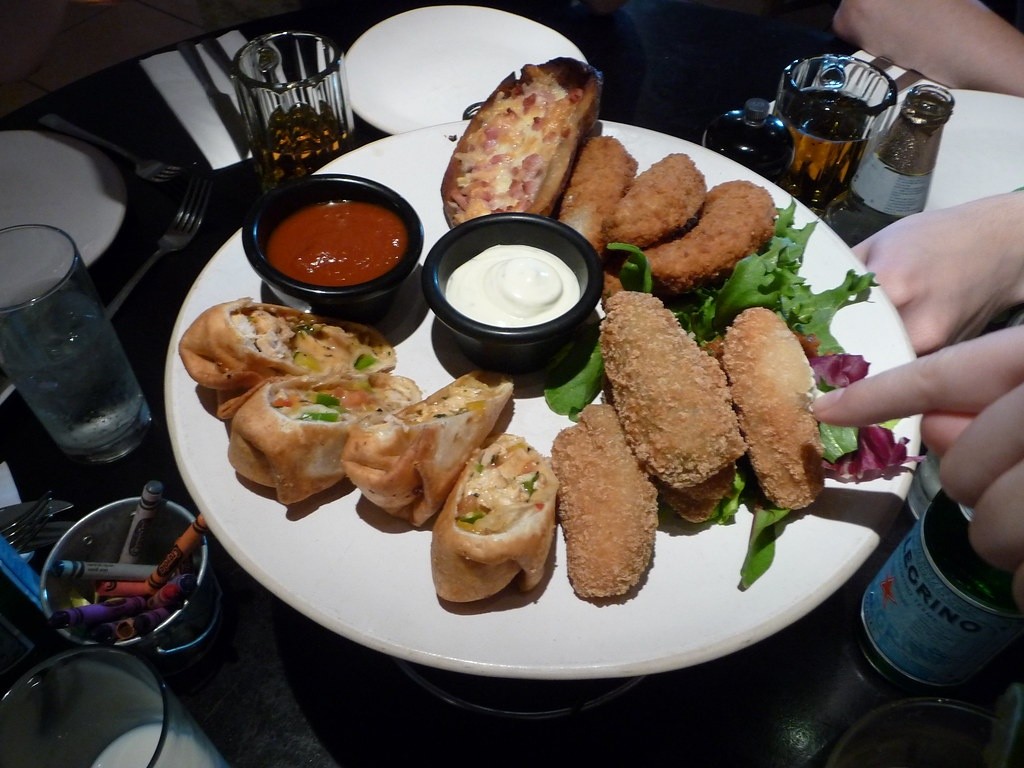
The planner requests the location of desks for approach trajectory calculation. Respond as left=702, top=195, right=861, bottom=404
left=0, top=0, right=916, bottom=768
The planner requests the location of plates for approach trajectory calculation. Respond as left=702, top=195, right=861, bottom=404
left=163, top=120, right=923, bottom=680
left=0, top=131, right=127, bottom=308
left=341, top=5, right=589, bottom=135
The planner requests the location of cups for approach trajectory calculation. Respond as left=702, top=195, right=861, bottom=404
left=0, top=649, right=231, bottom=768
left=1, top=224, right=153, bottom=464
left=773, top=55, right=898, bottom=218
left=41, top=497, right=222, bottom=678
left=907, top=451, right=941, bottom=520
left=823, top=696, right=1024, bottom=768
left=232, top=29, right=356, bottom=195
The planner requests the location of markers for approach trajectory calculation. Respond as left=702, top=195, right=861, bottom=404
left=53, top=559, right=157, bottom=580
left=98, top=582, right=155, bottom=598
left=120, top=480, right=164, bottom=564
left=51, top=573, right=198, bottom=644
left=148, top=512, right=209, bottom=587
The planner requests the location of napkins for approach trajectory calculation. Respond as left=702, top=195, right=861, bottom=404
left=138, top=26, right=254, bottom=171
left=838, top=47, right=1024, bottom=209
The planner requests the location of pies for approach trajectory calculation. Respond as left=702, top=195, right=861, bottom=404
left=178, top=297, right=556, bottom=602
left=441, top=56, right=603, bottom=222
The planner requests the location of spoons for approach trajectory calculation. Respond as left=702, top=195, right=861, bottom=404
left=0, top=499, right=74, bottom=528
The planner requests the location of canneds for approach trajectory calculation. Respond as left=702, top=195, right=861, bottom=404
left=861, top=485, right=1023, bottom=686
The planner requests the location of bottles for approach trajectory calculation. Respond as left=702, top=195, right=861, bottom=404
left=856, top=487, right=1024, bottom=702
left=702, top=99, right=795, bottom=181
left=818, top=84, right=954, bottom=249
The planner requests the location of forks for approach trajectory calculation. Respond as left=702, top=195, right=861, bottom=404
left=63, top=176, right=214, bottom=358
left=0, top=491, right=53, bottom=552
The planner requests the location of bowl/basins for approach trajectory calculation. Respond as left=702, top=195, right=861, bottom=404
left=242, top=174, right=424, bottom=325
left=423, top=211, right=602, bottom=371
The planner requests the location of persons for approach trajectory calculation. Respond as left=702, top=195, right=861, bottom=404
left=808, top=187, right=1024, bottom=611
left=831, top=0, right=1024, bottom=98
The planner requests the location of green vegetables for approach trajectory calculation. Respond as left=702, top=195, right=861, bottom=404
left=542, top=189, right=930, bottom=592
